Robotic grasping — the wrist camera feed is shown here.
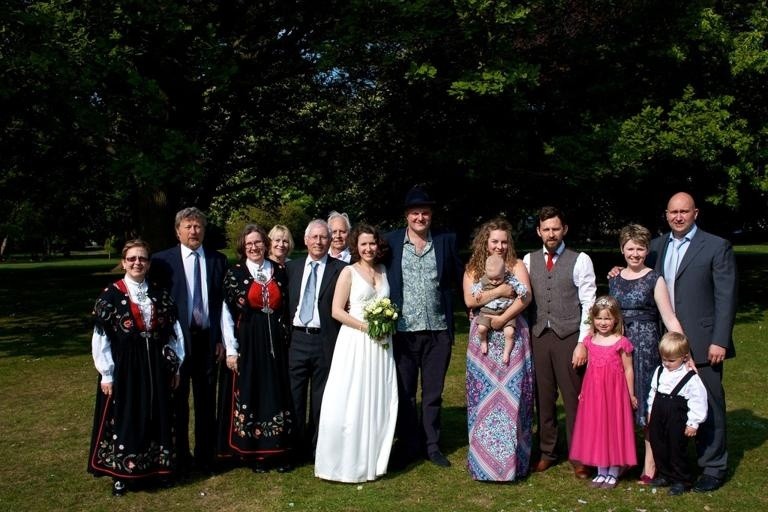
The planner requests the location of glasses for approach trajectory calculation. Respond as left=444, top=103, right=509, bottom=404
left=243, top=240, right=263, bottom=246
left=125, top=255, right=147, bottom=263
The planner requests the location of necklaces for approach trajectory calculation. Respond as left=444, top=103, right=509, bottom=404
left=364, top=268, right=377, bottom=285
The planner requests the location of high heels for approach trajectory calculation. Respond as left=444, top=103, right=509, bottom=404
left=638, top=467, right=658, bottom=485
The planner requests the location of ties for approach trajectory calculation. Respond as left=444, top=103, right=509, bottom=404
left=190, top=253, right=204, bottom=333
left=299, top=262, right=318, bottom=324
left=668, top=240, right=682, bottom=310
left=545, top=252, right=552, bottom=270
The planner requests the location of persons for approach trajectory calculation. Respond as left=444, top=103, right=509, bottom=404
left=218, top=218, right=297, bottom=475
left=276, top=218, right=350, bottom=457
left=323, top=208, right=353, bottom=266
left=462, top=217, right=535, bottom=482
left=144, top=206, right=231, bottom=474
left=566, top=295, right=639, bottom=489
left=642, top=189, right=736, bottom=489
left=473, top=254, right=528, bottom=367
left=606, top=220, right=701, bottom=487
left=264, top=220, right=294, bottom=265
left=519, top=204, right=597, bottom=482
left=378, top=189, right=472, bottom=471
left=89, top=237, right=186, bottom=500
left=313, top=226, right=395, bottom=484
left=644, top=332, right=710, bottom=498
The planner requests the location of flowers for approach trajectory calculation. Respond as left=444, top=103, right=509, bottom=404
left=363, top=296, right=399, bottom=350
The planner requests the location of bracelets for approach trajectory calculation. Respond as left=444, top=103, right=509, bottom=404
left=359, top=322, right=366, bottom=332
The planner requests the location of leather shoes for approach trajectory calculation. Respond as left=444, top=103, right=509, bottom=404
left=600, top=474, right=618, bottom=490
left=667, top=480, right=692, bottom=497
left=589, top=474, right=605, bottom=490
left=275, top=455, right=290, bottom=473
left=532, top=457, right=549, bottom=472
left=426, top=450, right=451, bottom=468
left=693, top=472, right=723, bottom=494
left=253, top=457, right=268, bottom=474
